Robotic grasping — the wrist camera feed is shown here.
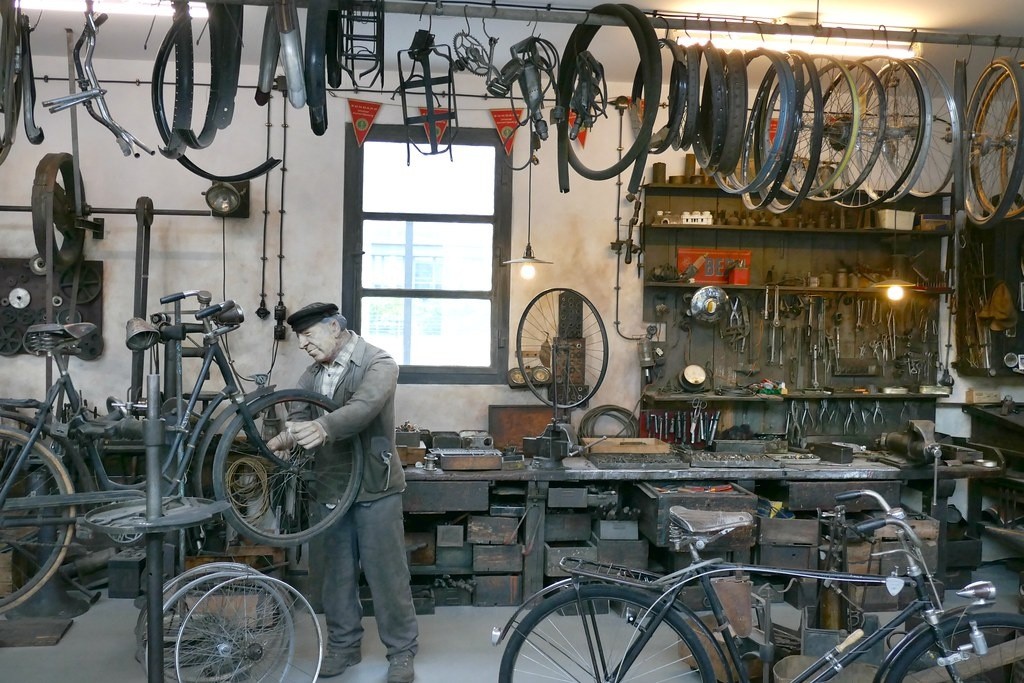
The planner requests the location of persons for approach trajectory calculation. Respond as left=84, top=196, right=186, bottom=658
left=266, top=302, right=419, bottom=683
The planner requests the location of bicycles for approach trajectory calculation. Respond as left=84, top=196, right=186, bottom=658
left=0, top=290, right=365, bottom=615
left=491, top=488, right=1024, bottom=683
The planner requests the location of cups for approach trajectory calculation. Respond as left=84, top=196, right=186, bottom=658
left=808, top=277, right=818, bottom=287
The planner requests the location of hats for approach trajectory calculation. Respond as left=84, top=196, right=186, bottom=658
left=285, top=302, right=339, bottom=332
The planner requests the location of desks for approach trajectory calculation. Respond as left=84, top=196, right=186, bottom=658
left=406, top=441, right=1005, bottom=609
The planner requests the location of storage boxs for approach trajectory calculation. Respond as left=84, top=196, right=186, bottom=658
left=393, top=428, right=1024, bottom=683
left=728, top=268, right=751, bottom=286
left=876, top=209, right=915, bottom=230
left=913, top=214, right=953, bottom=231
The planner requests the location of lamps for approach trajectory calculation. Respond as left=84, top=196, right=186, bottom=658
left=871, top=77, right=917, bottom=300
left=202, top=182, right=251, bottom=218
left=503, top=115, right=554, bottom=279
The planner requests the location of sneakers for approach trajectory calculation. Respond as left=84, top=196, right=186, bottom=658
left=386, top=653, right=416, bottom=683
left=317, top=646, right=362, bottom=678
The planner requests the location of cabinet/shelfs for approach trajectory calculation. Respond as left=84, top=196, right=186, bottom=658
left=961, top=403, right=1024, bottom=564
left=641, top=183, right=954, bottom=402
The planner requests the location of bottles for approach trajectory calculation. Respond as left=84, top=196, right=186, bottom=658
left=681, top=212, right=691, bottom=224
left=830, top=208, right=835, bottom=229
left=691, top=211, right=702, bottom=224
left=820, top=271, right=833, bottom=288
left=702, top=211, right=713, bottom=225
left=806, top=209, right=816, bottom=228
